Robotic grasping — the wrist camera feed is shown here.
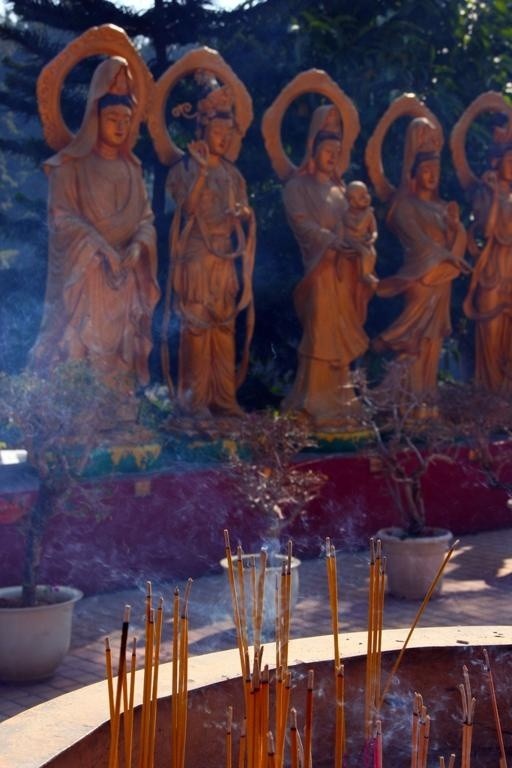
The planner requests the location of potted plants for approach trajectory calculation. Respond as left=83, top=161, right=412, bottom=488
left=1, top=364, right=177, bottom=683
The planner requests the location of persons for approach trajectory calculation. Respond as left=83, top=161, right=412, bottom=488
left=385, top=114, right=475, bottom=420
left=161, top=81, right=259, bottom=425
left=24, top=53, right=162, bottom=440
left=331, top=179, right=381, bottom=287
left=471, top=120, right=512, bottom=415
left=279, top=105, right=381, bottom=426
